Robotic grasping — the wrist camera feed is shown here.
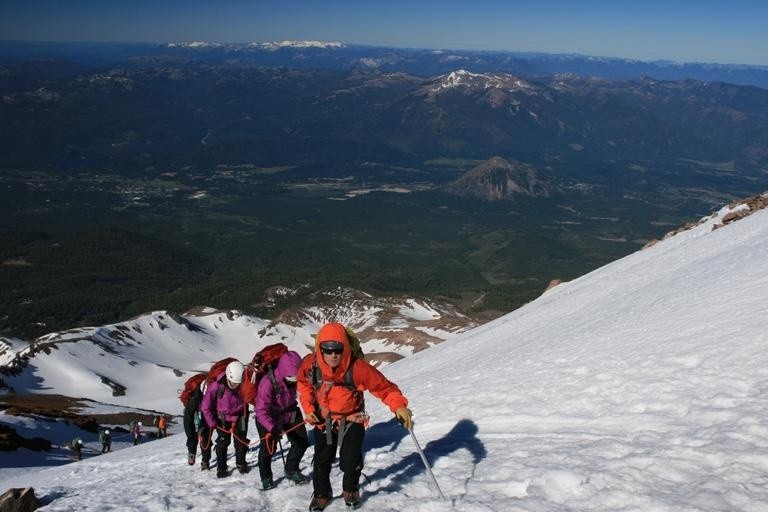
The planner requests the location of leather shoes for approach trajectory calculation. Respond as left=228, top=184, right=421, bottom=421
left=271, top=425, right=282, bottom=441
left=306, top=413, right=319, bottom=423
left=397, top=408, right=413, bottom=429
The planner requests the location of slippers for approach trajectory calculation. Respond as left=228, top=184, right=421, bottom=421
left=237, top=343, right=289, bottom=407
left=153, top=415, right=160, bottom=425
left=206, top=357, right=239, bottom=387
left=71, top=437, right=79, bottom=448
left=180, top=373, right=209, bottom=407
left=98, top=431, right=104, bottom=444
left=130, top=420, right=136, bottom=434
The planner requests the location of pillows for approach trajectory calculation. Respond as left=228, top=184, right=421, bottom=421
left=78, top=440, right=82, bottom=444
left=138, top=422, right=142, bottom=426
left=105, top=430, right=109, bottom=435
left=200, top=380, right=207, bottom=394
left=321, top=341, right=342, bottom=350
left=226, top=361, right=245, bottom=384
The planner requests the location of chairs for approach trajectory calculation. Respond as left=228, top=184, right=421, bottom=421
left=263, top=479, right=275, bottom=490
left=286, top=471, right=310, bottom=484
left=309, top=489, right=333, bottom=512
left=342, top=487, right=360, bottom=508
left=188, top=452, right=251, bottom=477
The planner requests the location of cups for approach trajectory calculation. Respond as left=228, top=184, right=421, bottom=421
left=320, top=346, right=343, bottom=355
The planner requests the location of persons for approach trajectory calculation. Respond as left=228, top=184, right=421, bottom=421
left=295, top=322, right=415, bottom=511
left=254, top=350, right=311, bottom=490
left=99, top=429, right=113, bottom=455
left=72, top=439, right=87, bottom=462
left=132, top=420, right=143, bottom=446
left=200, top=361, right=251, bottom=478
left=158, top=415, right=168, bottom=439
left=181, top=375, right=215, bottom=472
